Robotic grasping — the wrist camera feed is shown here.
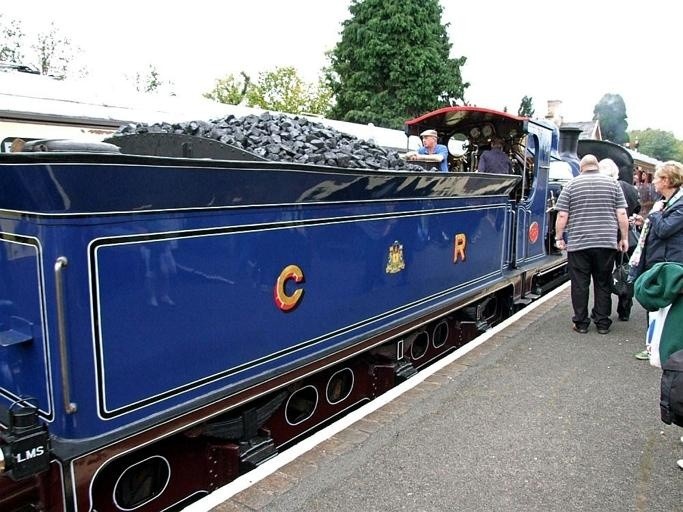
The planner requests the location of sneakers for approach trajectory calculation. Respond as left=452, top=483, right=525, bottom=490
left=572, top=323, right=589, bottom=333
left=634, top=347, right=651, bottom=360
left=618, top=314, right=630, bottom=322
left=596, top=326, right=610, bottom=334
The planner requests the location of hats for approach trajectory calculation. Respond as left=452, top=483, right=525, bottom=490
left=419, top=128, right=438, bottom=138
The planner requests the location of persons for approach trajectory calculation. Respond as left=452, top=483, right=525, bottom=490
left=404, top=130, right=449, bottom=173
left=626, top=160, right=683, bottom=361
left=553, top=154, right=630, bottom=335
left=476, top=136, right=512, bottom=174
left=598, top=158, right=682, bottom=321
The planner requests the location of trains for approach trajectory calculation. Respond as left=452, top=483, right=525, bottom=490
left=0, top=104, right=661, bottom=510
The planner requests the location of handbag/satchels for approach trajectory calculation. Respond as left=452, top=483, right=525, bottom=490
left=659, top=348, right=682, bottom=429
left=609, top=246, right=635, bottom=299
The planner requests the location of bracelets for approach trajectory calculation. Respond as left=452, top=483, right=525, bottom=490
left=554, top=236, right=565, bottom=240
